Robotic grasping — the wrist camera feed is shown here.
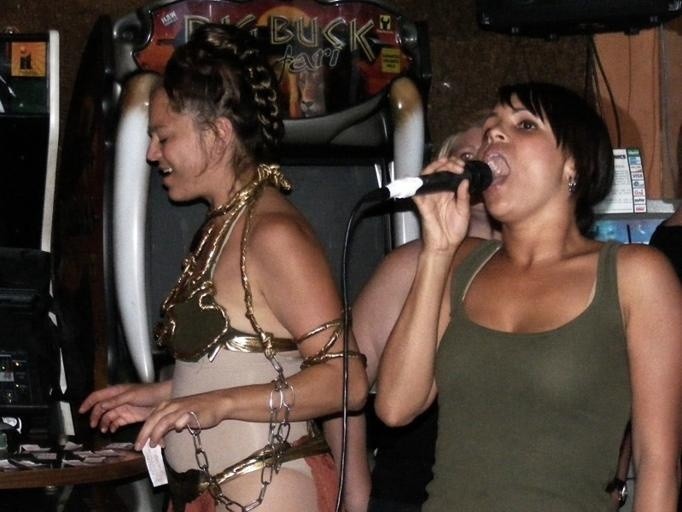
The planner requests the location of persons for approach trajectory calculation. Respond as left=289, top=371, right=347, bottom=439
left=323, top=113, right=502, bottom=511
left=606, top=419, right=633, bottom=507
left=649, top=118, right=682, bottom=512
left=79, top=23, right=368, bottom=512
left=373, top=82, right=682, bottom=512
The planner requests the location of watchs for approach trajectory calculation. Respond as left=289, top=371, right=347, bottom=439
left=612, top=478, right=625, bottom=497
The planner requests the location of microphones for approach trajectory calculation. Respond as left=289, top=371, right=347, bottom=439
left=351, top=160, right=493, bottom=209
